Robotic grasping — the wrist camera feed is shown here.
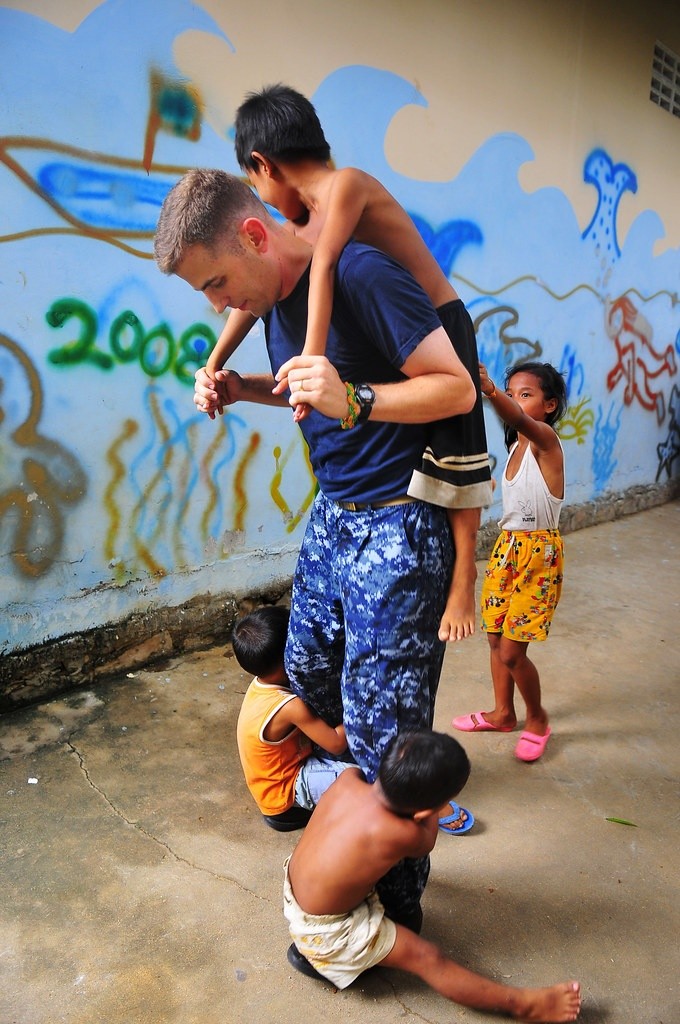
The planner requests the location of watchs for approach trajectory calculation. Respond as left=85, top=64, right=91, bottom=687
left=354, top=382, right=376, bottom=423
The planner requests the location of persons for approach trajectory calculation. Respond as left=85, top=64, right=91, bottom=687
left=452, top=361, right=568, bottom=761
left=156, top=170, right=477, bottom=981
left=281, top=730, right=582, bottom=1023
left=203, top=82, right=496, bottom=643
left=234, top=607, right=476, bottom=835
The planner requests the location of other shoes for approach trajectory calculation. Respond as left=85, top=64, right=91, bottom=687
left=263, top=807, right=313, bottom=833
left=286, top=942, right=325, bottom=981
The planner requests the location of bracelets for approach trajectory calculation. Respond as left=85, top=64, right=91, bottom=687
left=484, top=378, right=496, bottom=399
left=341, top=381, right=361, bottom=429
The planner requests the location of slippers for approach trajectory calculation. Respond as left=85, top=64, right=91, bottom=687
left=514, top=725, right=553, bottom=761
left=451, top=710, right=513, bottom=732
left=435, top=801, right=475, bottom=834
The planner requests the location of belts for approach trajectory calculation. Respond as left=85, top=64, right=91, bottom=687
left=333, top=493, right=417, bottom=511
